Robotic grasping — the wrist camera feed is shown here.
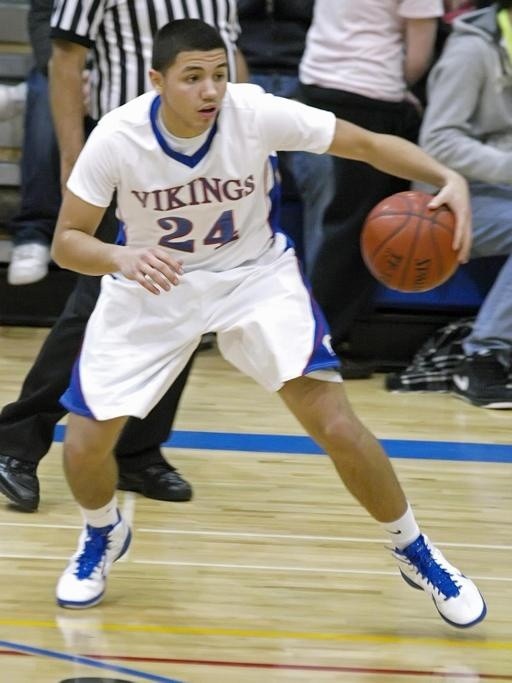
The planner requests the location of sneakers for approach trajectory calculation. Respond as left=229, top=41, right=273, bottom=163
left=448, top=350, right=512, bottom=410
left=6, top=243, right=51, bottom=286
left=55, top=507, right=131, bottom=609
left=383, top=533, right=486, bottom=629
left=334, top=341, right=379, bottom=379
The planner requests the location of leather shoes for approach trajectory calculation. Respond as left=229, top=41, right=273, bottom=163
left=116, top=461, right=191, bottom=501
left=0, top=454, right=39, bottom=513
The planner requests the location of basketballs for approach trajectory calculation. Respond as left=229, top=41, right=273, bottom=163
left=361, top=192, right=460, bottom=294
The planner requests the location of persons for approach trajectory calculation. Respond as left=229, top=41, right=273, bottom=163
left=1, top=0, right=252, bottom=510
left=52, top=18, right=485, bottom=626
left=9, top=0, right=510, bottom=408
